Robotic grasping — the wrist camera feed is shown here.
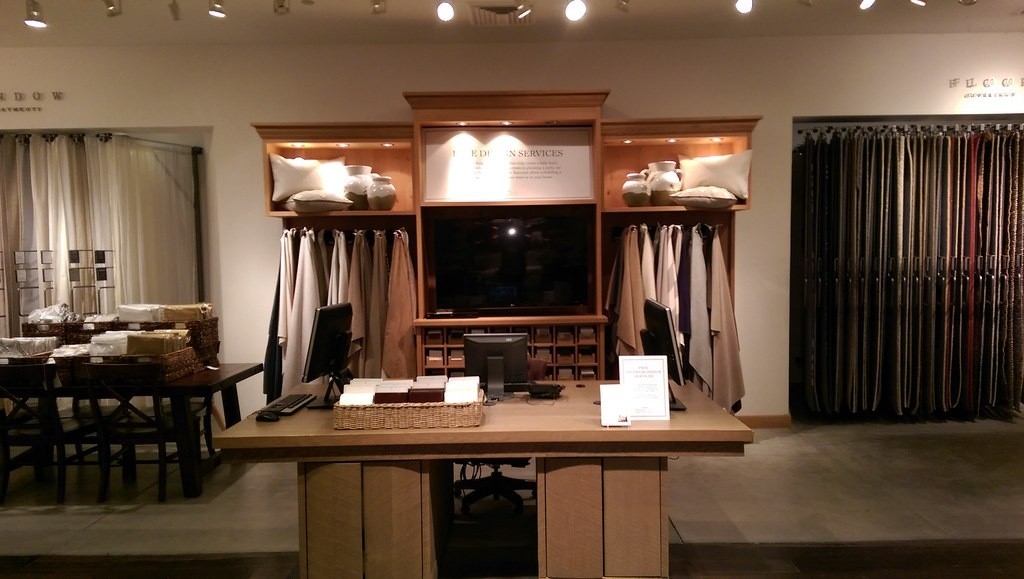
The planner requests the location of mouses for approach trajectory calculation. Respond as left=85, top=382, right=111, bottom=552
left=256, top=412, right=279, bottom=422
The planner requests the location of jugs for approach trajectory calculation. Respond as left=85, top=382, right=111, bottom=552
left=642, top=160, right=683, bottom=205
left=345, top=165, right=380, bottom=209
left=367, top=176, right=396, bottom=210
left=623, top=173, right=650, bottom=207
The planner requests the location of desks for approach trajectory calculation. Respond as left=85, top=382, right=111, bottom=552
left=212, top=380, right=754, bottom=579
left=0, top=363, right=264, bottom=498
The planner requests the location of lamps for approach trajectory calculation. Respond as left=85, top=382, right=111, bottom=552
left=368, top=0, right=386, bottom=15
left=103, top=0, right=123, bottom=17
left=168, top=0, right=181, bottom=21
left=24, top=0, right=47, bottom=28
left=273, top=0, right=290, bottom=16
left=208, top=0, right=226, bottom=18
left=513, top=0, right=532, bottom=19
left=615, top=0, right=631, bottom=14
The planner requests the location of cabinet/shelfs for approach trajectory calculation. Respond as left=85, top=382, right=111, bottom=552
left=250, top=89, right=765, bottom=416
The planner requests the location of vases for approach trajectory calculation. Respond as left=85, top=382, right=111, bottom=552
left=639, top=161, right=684, bottom=206
left=367, top=176, right=396, bottom=211
left=344, top=164, right=381, bottom=210
left=622, top=173, right=651, bottom=207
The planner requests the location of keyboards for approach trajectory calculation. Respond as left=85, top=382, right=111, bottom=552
left=480, top=382, right=558, bottom=392
left=258, top=393, right=317, bottom=416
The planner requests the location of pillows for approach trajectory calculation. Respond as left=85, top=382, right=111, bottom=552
left=275, top=189, right=354, bottom=211
left=666, top=186, right=740, bottom=208
left=268, top=153, right=345, bottom=202
left=677, top=149, right=753, bottom=200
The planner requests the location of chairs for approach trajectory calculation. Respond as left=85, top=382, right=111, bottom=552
left=453, top=358, right=547, bottom=515
left=0, top=341, right=222, bottom=504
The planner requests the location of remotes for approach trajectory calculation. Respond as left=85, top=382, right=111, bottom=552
left=426, top=310, right=481, bottom=319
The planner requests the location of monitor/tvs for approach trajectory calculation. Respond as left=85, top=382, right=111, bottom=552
left=433, top=214, right=589, bottom=316
left=301, top=302, right=354, bottom=409
left=463, top=332, right=529, bottom=402
left=639, top=297, right=687, bottom=411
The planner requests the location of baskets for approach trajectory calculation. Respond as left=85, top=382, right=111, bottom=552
left=0, top=317, right=221, bottom=384
left=332, top=388, right=484, bottom=429
